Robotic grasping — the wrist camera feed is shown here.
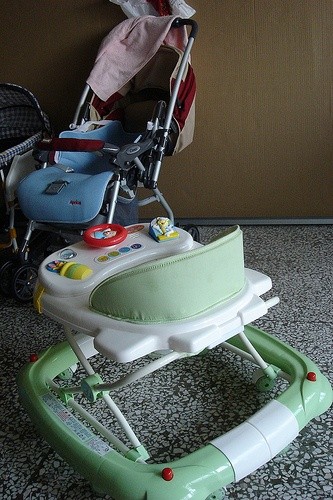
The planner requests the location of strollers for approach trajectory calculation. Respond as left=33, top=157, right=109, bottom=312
left=18, top=218, right=333, bottom=500
left=0, top=82, right=53, bottom=296
left=13, top=18, right=201, bottom=302
